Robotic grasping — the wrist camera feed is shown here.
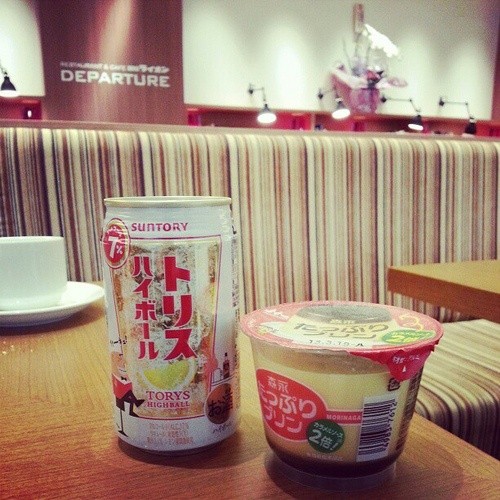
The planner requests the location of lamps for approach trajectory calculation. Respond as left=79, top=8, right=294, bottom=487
left=318, top=85, right=350, bottom=121
left=439, top=97, right=477, bottom=138
left=248, top=87, right=277, bottom=126
left=0, top=62, right=16, bottom=97
left=380, top=95, right=424, bottom=131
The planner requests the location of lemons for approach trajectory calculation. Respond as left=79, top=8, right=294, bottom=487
left=138, top=355, right=196, bottom=392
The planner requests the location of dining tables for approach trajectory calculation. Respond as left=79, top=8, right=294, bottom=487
left=0, top=281, right=500, bottom=500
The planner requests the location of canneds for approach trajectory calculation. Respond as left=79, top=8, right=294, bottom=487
left=98, top=195, right=242, bottom=452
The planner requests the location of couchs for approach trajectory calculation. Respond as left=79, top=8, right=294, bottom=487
left=0, top=119, right=500, bottom=454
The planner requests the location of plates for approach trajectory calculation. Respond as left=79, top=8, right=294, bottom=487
left=1, top=281, right=105, bottom=328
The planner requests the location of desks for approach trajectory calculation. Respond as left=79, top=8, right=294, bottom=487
left=387, top=259, right=500, bottom=324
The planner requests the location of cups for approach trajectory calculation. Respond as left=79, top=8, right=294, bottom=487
left=0, top=236, right=67, bottom=310
